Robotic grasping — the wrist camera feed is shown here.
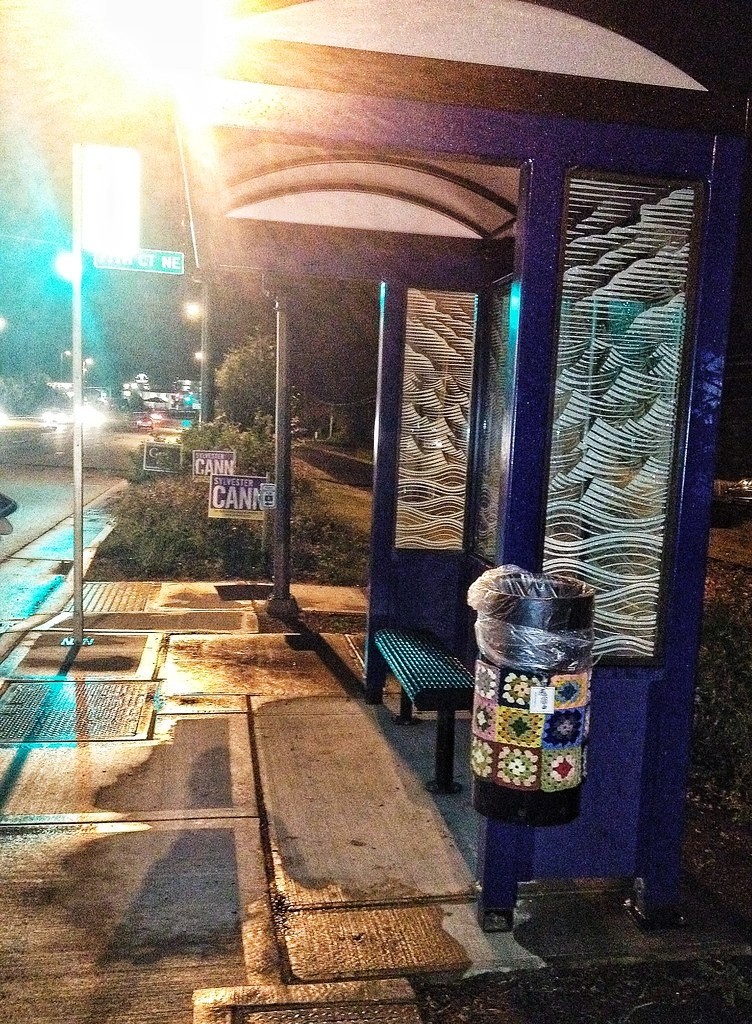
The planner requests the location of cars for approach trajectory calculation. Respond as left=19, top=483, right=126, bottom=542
left=712, top=448, right=752, bottom=510
left=137, top=417, right=154, bottom=430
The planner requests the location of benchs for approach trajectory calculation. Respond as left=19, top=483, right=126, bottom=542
left=373, top=626, right=474, bottom=796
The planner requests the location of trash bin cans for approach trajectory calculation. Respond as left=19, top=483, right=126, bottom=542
left=470, top=574, right=594, bottom=827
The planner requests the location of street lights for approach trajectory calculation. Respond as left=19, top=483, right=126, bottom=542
left=184, top=298, right=214, bottom=427
left=58, top=351, right=72, bottom=381
left=55, top=246, right=89, bottom=642
left=82, top=358, right=92, bottom=383
